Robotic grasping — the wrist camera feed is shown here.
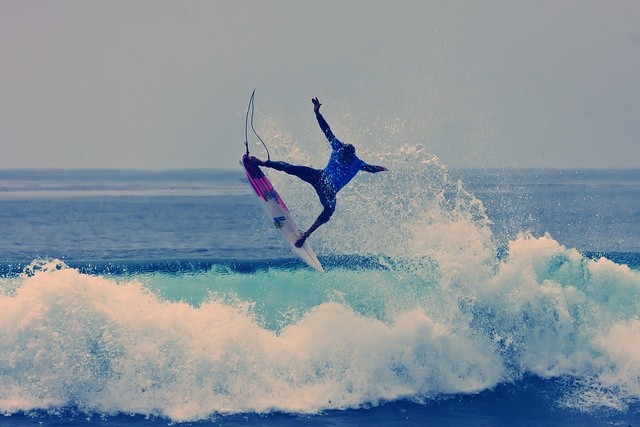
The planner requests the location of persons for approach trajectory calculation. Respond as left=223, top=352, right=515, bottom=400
left=251, top=97, right=389, bottom=248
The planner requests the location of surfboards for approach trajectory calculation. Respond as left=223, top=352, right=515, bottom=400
left=242, top=154, right=326, bottom=274
